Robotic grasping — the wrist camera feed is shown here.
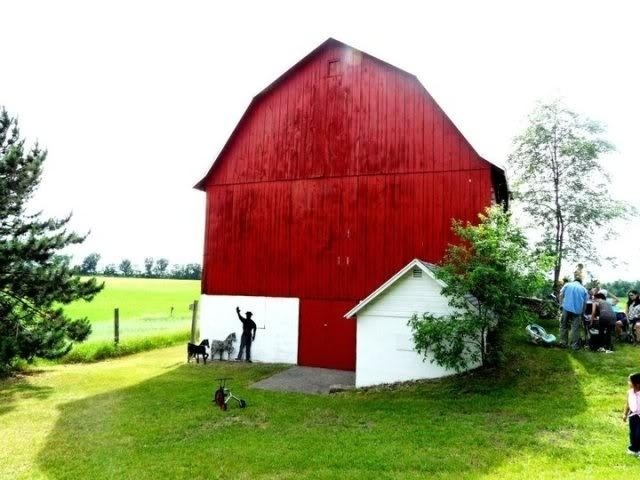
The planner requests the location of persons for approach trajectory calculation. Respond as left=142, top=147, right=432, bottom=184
left=235, top=307, right=256, bottom=364
left=623, top=373, right=640, bottom=454
left=552, top=263, right=640, bottom=353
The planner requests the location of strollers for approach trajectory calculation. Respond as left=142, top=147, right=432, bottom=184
left=614, top=309, right=637, bottom=345
left=576, top=312, right=614, bottom=352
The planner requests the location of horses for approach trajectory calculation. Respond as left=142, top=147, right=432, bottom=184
left=211, top=332, right=237, bottom=361
left=188, top=339, right=210, bottom=365
